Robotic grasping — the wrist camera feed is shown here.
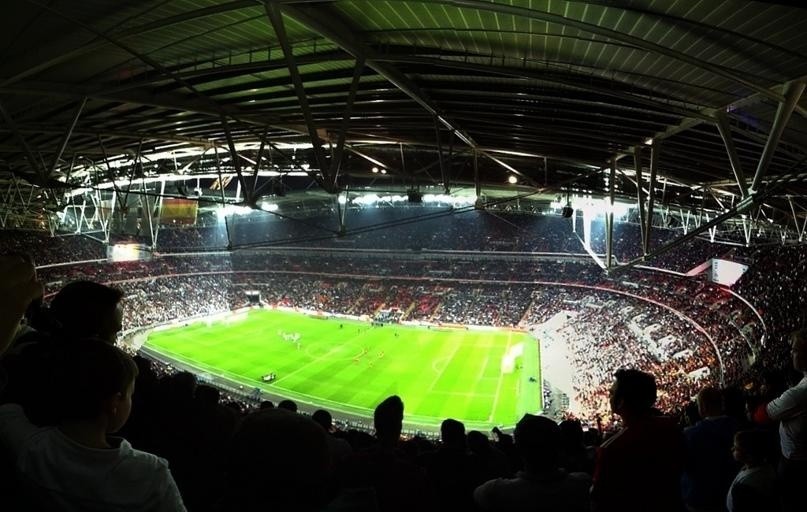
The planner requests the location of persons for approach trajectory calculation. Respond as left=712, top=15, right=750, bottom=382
left=0, top=201, right=807, bottom=512
